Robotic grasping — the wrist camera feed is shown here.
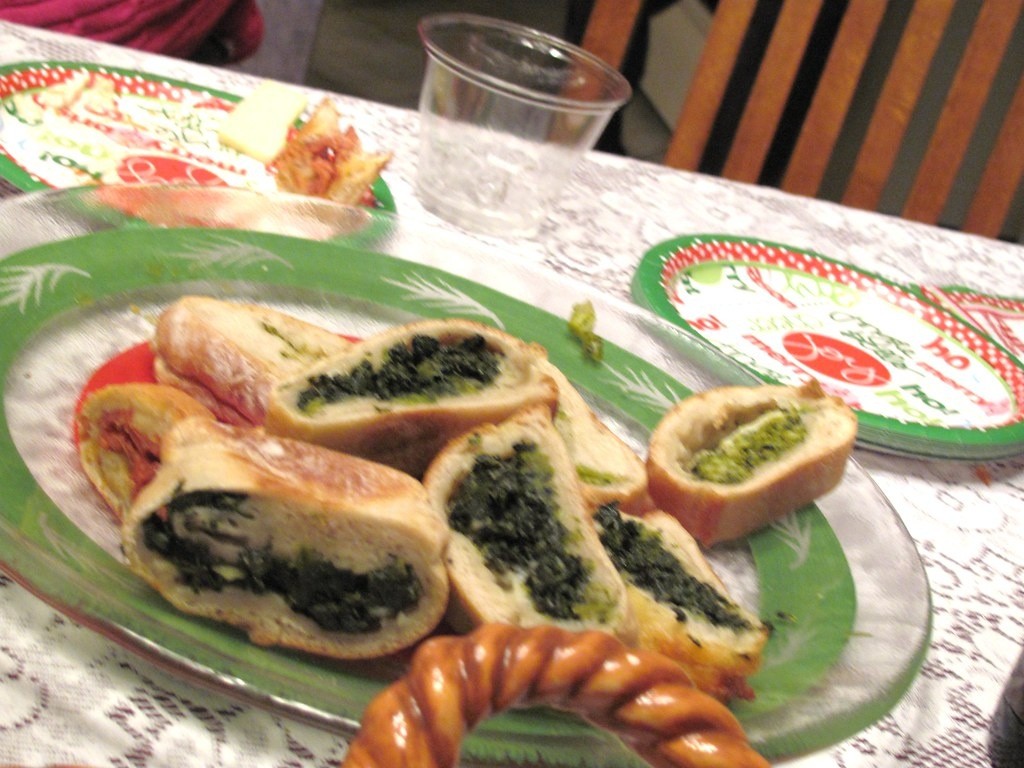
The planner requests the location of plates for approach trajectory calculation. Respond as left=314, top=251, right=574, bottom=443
left=0, top=61, right=398, bottom=262
left=629, top=232, right=1024, bottom=464
left=0, top=187, right=934, bottom=760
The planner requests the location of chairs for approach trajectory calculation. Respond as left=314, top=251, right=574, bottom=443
left=542, top=1, right=1023, bottom=239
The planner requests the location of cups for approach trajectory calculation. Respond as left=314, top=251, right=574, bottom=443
left=411, top=10, right=633, bottom=243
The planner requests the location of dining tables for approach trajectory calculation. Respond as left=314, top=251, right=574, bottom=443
left=0, top=19, right=1024, bottom=768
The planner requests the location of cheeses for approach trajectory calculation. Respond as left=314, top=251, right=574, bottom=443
left=217, top=80, right=307, bottom=165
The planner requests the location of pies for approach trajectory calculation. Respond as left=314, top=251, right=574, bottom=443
left=73, top=294, right=861, bottom=706
